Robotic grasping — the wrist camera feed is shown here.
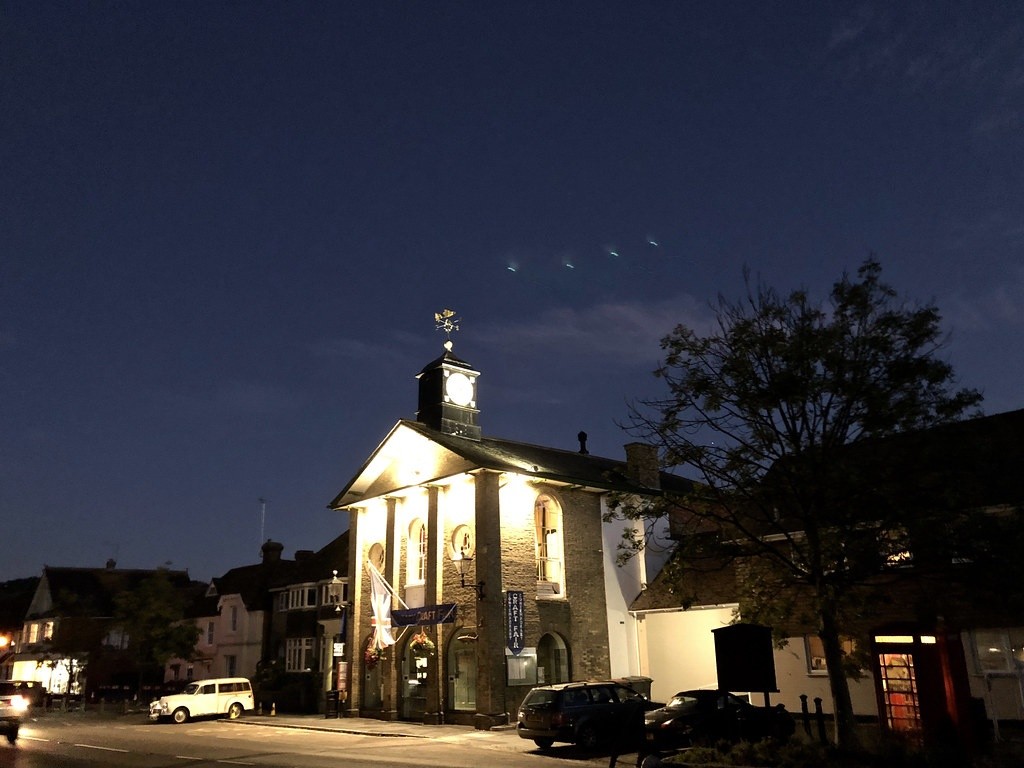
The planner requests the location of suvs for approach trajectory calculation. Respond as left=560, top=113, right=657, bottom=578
left=0, top=680, right=42, bottom=740
left=517, top=682, right=664, bottom=756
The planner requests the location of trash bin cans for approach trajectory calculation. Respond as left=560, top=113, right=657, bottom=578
left=623, top=676, right=653, bottom=702
left=607, top=678, right=628, bottom=702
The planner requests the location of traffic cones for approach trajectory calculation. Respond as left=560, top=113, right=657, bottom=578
left=257, top=701, right=264, bottom=715
left=267, top=702, right=277, bottom=717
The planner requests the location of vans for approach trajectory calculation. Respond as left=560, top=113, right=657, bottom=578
left=148, top=679, right=255, bottom=724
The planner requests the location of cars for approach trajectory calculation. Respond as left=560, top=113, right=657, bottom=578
left=643, top=688, right=796, bottom=750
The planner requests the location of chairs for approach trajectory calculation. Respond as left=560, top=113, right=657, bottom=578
left=571, top=693, right=587, bottom=702
left=597, top=693, right=610, bottom=703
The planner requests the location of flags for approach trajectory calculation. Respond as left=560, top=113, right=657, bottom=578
left=367, top=564, right=397, bottom=649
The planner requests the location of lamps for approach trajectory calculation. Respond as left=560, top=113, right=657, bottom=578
left=327, top=569, right=354, bottom=619
left=451, top=546, right=486, bottom=602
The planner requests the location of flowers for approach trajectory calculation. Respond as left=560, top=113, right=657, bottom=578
left=365, top=638, right=383, bottom=671
left=409, top=624, right=434, bottom=656
